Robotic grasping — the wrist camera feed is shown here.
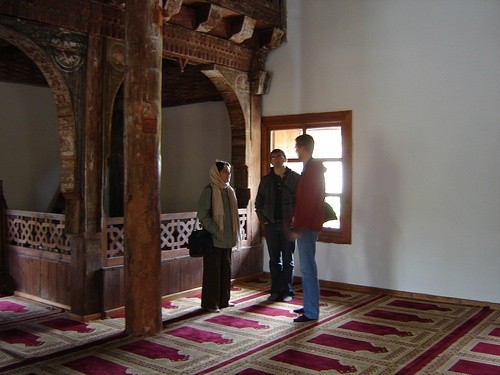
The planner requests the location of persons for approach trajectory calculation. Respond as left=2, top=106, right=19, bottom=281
left=293, top=135, right=327, bottom=323
left=255, top=149, right=301, bottom=301
left=198, top=161, right=241, bottom=313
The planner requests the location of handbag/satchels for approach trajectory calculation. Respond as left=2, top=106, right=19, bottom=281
left=188, top=229, right=214, bottom=258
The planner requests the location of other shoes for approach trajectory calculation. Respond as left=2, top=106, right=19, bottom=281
left=294, top=314, right=316, bottom=322
left=203, top=306, right=220, bottom=312
left=228, top=302, right=234, bottom=306
left=268, top=295, right=283, bottom=301
left=293, top=308, right=305, bottom=313
left=285, top=290, right=294, bottom=296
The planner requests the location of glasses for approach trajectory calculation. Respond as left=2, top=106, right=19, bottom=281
left=272, top=155, right=284, bottom=160
